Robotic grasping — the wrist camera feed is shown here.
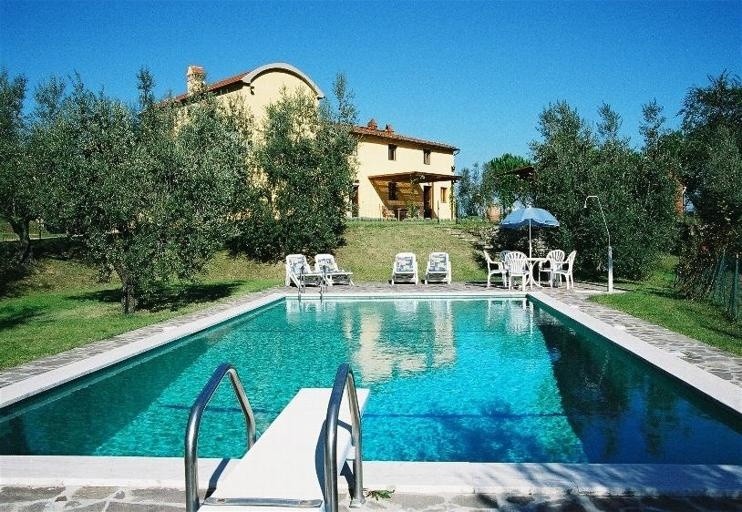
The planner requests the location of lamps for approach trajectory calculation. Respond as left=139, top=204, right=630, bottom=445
left=452, top=165, right=455, bottom=172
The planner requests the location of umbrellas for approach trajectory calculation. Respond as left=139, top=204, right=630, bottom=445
left=498, top=207, right=560, bottom=258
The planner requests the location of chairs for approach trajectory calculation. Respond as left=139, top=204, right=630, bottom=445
left=285, top=253, right=353, bottom=291
left=483, top=248, right=577, bottom=292
left=392, top=252, right=418, bottom=285
left=424, top=251, right=451, bottom=284
